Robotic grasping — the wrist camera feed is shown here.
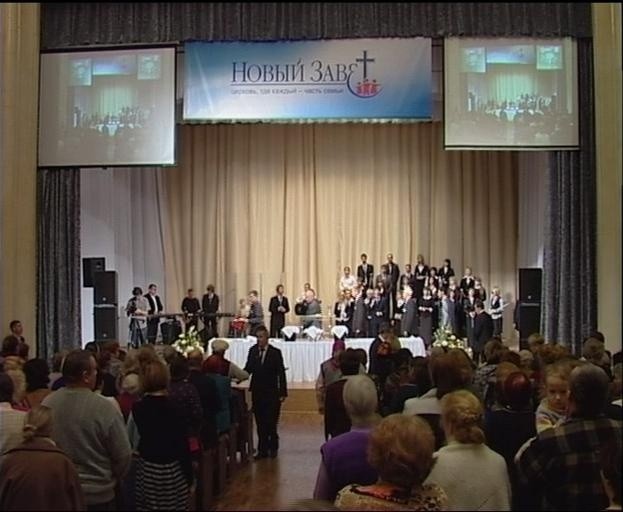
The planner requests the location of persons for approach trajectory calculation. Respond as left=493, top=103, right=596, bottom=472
left=340, top=253, right=504, bottom=365
left=125, top=283, right=219, bottom=348
left=235, top=283, right=322, bottom=338
left=67, top=103, right=151, bottom=138
left=474, top=92, right=558, bottom=144
left=244, top=327, right=287, bottom=460
left=1, top=320, right=248, bottom=512
left=316, top=323, right=623, bottom=512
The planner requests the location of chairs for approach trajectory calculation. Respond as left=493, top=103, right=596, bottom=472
left=0, top=345, right=257, bottom=509
left=315, top=348, right=621, bottom=511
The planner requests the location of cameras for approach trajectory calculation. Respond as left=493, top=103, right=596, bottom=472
left=128, top=301, right=137, bottom=313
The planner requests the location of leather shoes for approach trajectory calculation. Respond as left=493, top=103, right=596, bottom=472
left=270, top=446, right=277, bottom=457
left=254, top=452, right=267, bottom=458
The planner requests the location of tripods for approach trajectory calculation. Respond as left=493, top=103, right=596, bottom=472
left=129, top=314, right=144, bottom=350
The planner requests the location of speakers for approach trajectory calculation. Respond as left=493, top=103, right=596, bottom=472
left=82, top=257, right=106, bottom=288
left=94, top=270, right=118, bottom=307
left=94, top=307, right=119, bottom=341
left=519, top=305, right=541, bottom=340
left=519, top=339, right=529, bottom=351
left=518, top=267, right=542, bottom=305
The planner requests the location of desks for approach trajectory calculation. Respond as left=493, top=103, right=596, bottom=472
left=205, top=335, right=430, bottom=391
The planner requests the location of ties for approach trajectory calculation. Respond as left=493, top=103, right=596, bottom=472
left=259, top=348, right=266, bottom=362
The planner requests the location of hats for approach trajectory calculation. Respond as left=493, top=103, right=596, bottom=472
left=376, top=321, right=392, bottom=333
left=333, top=339, right=345, bottom=351
left=212, top=339, right=230, bottom=352
